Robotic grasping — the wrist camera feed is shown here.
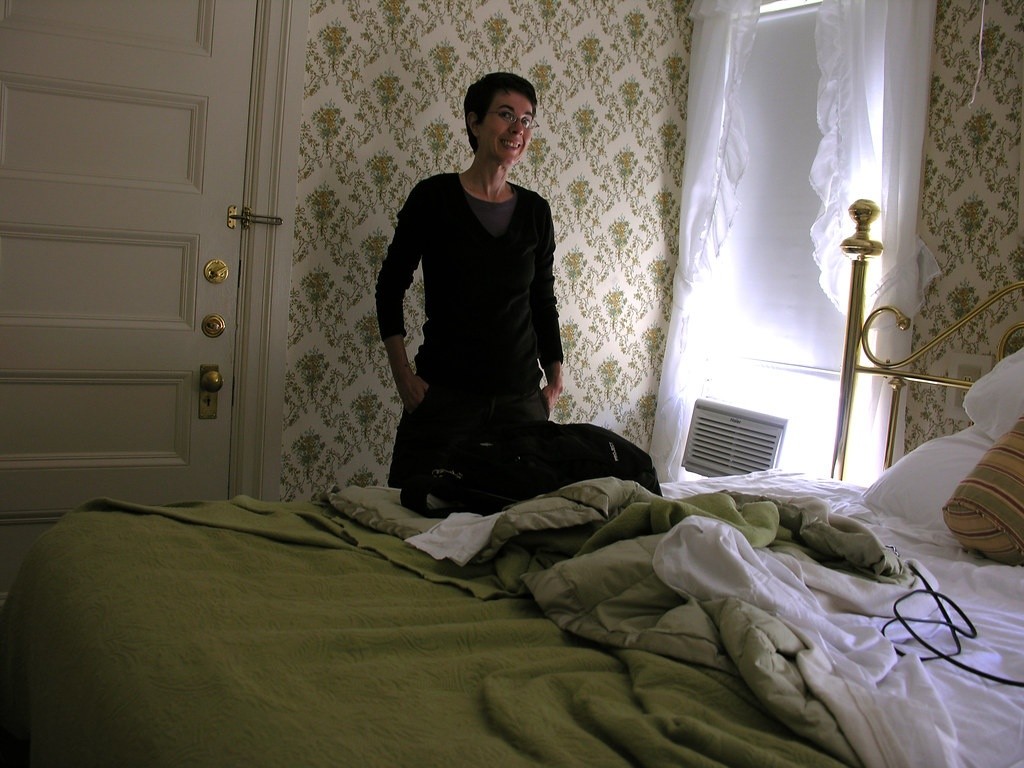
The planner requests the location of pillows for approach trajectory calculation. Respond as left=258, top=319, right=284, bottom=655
left=942, top=415, right=1024, bottom=567
left=861, top=423, right=996, bottom=548
left=961, top=347, right=1024, bottom=442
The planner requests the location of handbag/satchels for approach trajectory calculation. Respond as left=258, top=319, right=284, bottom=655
left=400, top=421, right=664, bottom=521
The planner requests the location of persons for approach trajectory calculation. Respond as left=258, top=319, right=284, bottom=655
left=378, top=73, right=565, bottom=489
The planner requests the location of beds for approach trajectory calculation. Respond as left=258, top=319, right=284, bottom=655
left=0, top=198, right=1024, bottom=768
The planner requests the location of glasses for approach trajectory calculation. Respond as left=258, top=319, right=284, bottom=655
left=485, top=109, right=540, bottom=130
